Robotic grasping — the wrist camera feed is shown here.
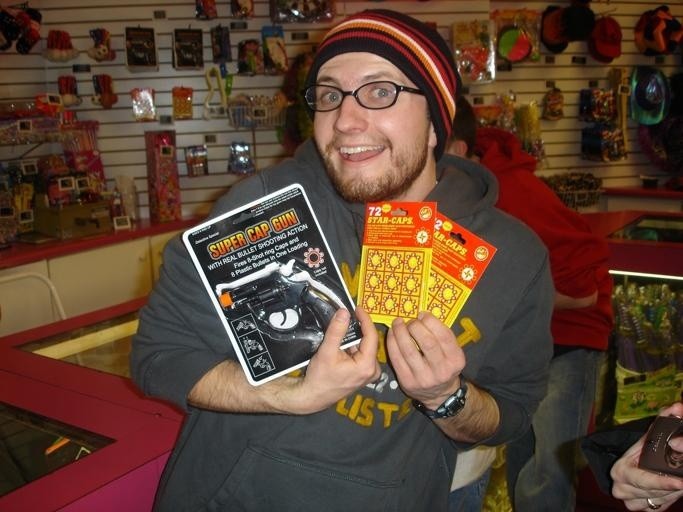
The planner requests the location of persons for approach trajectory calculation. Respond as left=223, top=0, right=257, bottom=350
left=240, top=39, right=262, bottom=72
left=446, top=97, right=617, bottom=512
left=128, top=9, right=555, bottom=512
left=608, top=402, right=683, bottom=512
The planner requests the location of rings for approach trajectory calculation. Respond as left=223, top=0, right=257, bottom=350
left=645, top=496, right=661, bottom=510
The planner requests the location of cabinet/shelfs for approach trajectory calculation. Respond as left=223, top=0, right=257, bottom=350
left=0, top=223, right=202, bottom=347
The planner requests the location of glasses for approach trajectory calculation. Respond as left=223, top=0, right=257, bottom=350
left=305, top=81, right=425, bottom=112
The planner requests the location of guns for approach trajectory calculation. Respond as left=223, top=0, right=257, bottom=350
left=214, top=271, right=350, bottom=337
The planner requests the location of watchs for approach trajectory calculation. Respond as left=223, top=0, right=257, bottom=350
left=409, top=373, right=469, bottom=419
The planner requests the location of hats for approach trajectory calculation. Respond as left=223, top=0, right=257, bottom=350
left=303, top=9, right=461, bottom=160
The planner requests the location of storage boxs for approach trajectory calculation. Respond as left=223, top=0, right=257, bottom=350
left=33, top=193, right=112, bottom=240
